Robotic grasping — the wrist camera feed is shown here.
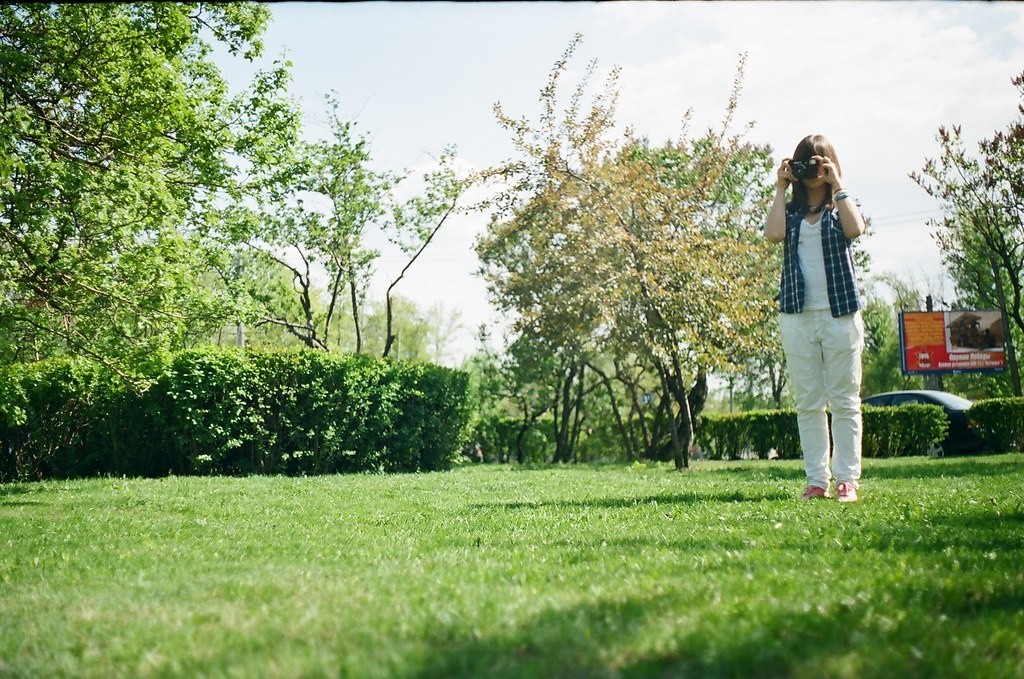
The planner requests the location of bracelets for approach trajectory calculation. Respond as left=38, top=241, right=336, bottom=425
left=834, top=188, right=849, bottom=201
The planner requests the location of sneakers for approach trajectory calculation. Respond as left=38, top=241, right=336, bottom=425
left=833, top=481, right=858, bottom=503
left=799, top=485, right=830, bottom=499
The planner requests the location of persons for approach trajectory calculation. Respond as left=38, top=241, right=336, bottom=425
left=764, top=135, right=866, bottom=503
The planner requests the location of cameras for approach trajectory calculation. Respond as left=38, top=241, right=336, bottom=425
left=788, top=159, right=817, bottom=179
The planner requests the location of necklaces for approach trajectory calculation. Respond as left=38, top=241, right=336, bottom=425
left=810, top=201, right=824, bottom=209
